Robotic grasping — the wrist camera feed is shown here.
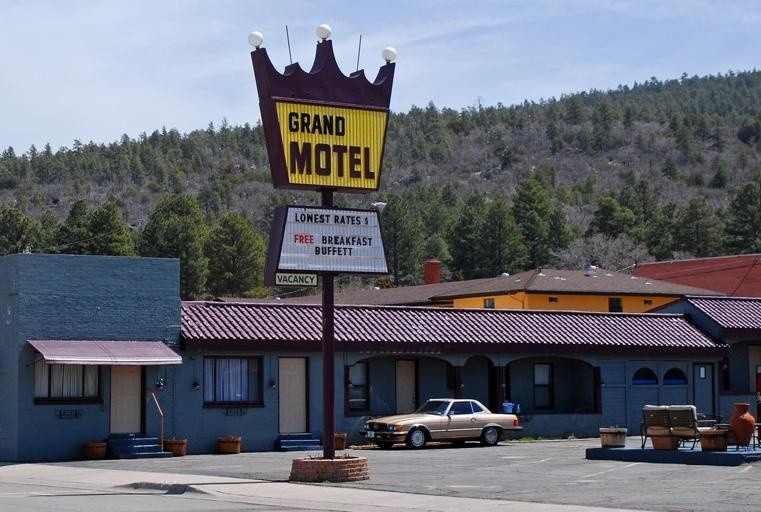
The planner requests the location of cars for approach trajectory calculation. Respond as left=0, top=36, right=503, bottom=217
left=358, top=397, right=524, bottom=452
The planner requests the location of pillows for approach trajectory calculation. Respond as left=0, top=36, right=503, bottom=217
left=645, top=404, right=697, bottom=429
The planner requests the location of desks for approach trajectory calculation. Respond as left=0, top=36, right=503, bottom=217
left=714, top=423, right=761, bottom=451
left=698, top=420, right=716, bottom=427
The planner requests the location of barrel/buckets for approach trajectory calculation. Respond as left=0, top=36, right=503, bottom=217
left=334, top=432, right=345, bottom=448
left=82, top=442, right=109, bottom=460
left=218, top=436, right=239, bottom=453
left=165, top=439, right=187, bottom=456
left=599, top=428, right=627, bottom=448
left=700, top=429, right=728, bottom=452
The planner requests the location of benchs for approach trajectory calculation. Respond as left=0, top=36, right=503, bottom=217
left=640, top=408, right=703, bottom=450
